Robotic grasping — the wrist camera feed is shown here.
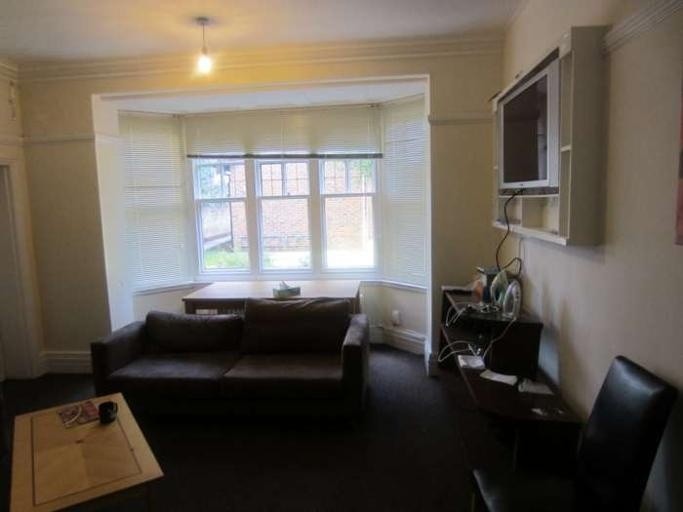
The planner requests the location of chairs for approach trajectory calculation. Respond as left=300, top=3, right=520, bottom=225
left=468, top=354, right=678, bottom=512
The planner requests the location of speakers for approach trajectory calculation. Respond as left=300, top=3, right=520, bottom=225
left=487, top=320, right=544, bottom=382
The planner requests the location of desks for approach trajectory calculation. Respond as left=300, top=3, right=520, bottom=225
left=9, top=392, right=165, bottom=512
left=181, top=280, right=363, bottom=319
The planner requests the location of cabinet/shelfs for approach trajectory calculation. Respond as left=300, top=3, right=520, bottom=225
left=437, top=288, right=582, bottom=469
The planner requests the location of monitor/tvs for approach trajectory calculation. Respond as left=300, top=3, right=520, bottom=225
left=496, top=56, right=561, bottom=196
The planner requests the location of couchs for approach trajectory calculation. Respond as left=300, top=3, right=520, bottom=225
left=89, top=296, right=368, bottom=429
